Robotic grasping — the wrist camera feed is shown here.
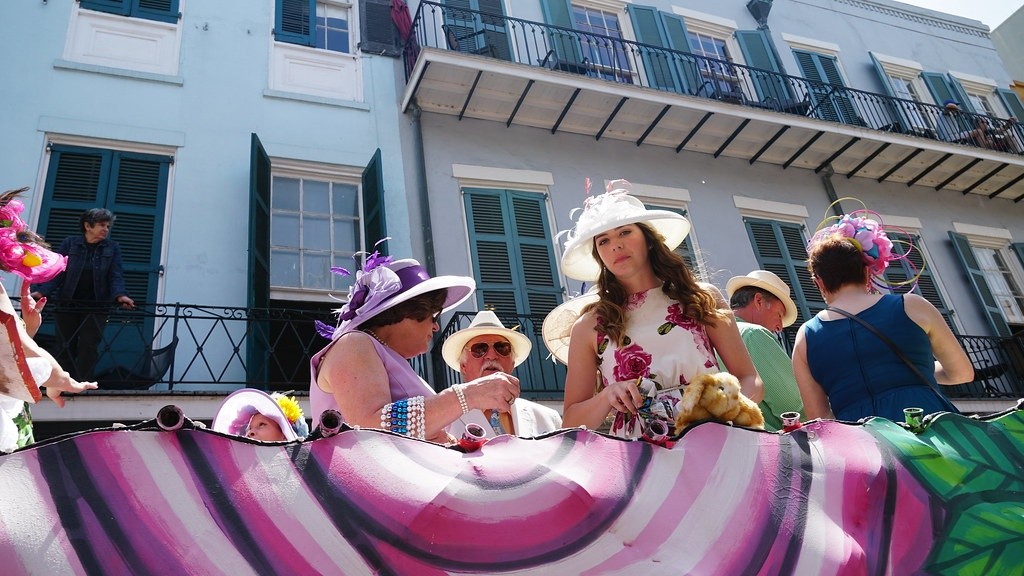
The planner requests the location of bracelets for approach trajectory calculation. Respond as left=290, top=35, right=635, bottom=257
left=452, top=383, right=469, bottom=415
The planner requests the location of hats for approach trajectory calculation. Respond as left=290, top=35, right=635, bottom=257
left=725, top=270, right=797, bottom=327
left=314, top=236, right=474, bottom=341
left=442, top=311, right=532, bottom=373
left=0, top=185, right=69, bottom=282
left=541, top=283, right=602, bottom=366
left=554, top=179, right=692, bottom=282
left=210, top=387, right=309, bottom=443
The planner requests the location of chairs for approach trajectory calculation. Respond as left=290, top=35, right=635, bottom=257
left=971, top=360, right=1014, bottom=399
left=95, top=336, right=180, bottom=391
left=857, top=115, right=1013, bottom=153
left=537, top=50, right=591, bottom=76
left=441, top=25, right=499, bottom=58
left=688, top=81, right=816, bottom=118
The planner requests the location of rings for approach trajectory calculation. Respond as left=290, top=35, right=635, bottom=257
left=508, top=397, right=515, bottom=405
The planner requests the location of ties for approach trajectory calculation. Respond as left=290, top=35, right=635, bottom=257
left=490, top=408, right=506, bottom=437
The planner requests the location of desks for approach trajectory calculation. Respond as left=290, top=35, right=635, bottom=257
left=585, top=62, right=637, bottom=83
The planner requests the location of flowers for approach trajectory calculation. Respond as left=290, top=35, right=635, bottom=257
left=316, top=237, right=403, bottom=341
left=0, top=186, right=52, bottom=269
left=552, top=176, right=645, bottom=250
left=825, top=215, right=894, bottom=281
left=271, top=391, right=309, bottom=439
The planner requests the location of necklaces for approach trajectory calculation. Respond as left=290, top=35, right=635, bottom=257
left=381, top=395, right=426, bottom=442
left=363, top=328, right=389, bottom=348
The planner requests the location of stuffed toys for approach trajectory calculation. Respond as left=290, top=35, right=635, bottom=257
left=674, top=372, right=765, bottom=435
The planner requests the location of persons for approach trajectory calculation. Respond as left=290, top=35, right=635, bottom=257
left=936, top=100, right=1024, bottom=156
left=712, top=268, right=805, bottom=432
left=0, top=282, right=98, bottom=454
left=441, top=311, right=563, bottom=440
left=562, top=177, right=765, bottom=441
left=792, top=197, right=975, bottom=425
left=542, top=282, right=620, bottom=424
left=310, top=237, right=519, bottom=448
left=29, top=207, right=135, bottom=382
left=211, top=387, right=310, bottom=444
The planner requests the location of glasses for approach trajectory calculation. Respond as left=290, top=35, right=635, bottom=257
left=464, top=341, right=511, bottom=358
left=433, top=304, right=444, bottom=323
